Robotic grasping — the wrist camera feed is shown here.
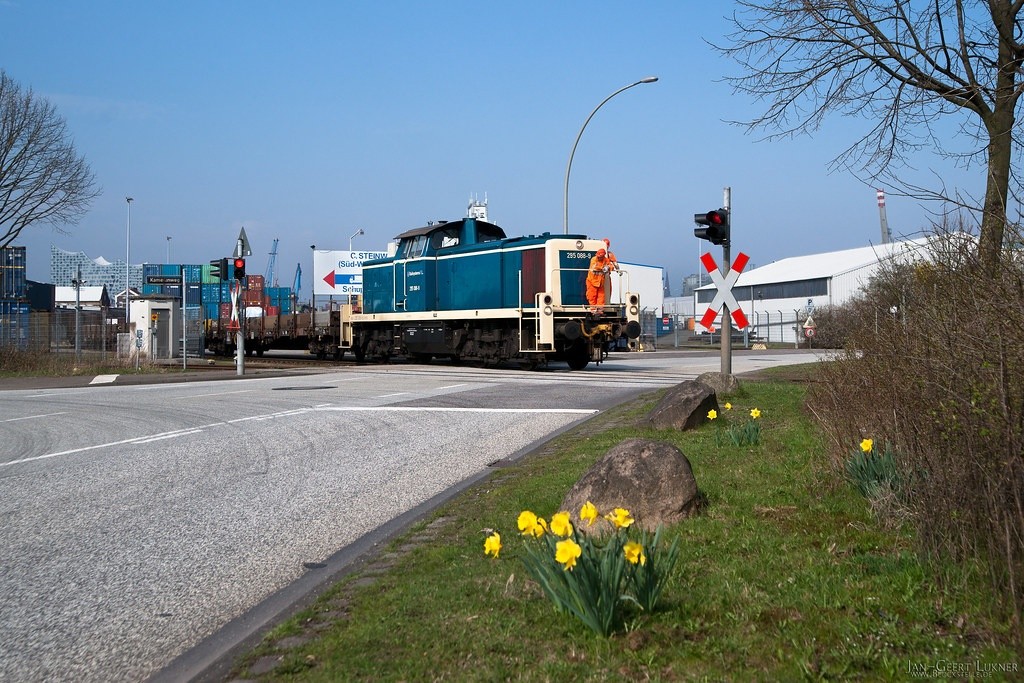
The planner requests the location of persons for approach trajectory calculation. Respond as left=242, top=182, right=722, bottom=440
left=601, top=238, right=623, bottom=298
left=586, top=249, right=614, bottom=316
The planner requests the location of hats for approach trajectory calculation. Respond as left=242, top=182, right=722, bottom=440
left=602, top=238, right=610, bottom=248
left=596, top=249, right=606, bottom=257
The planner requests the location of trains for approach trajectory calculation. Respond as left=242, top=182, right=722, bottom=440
left=201, top=193, right=645, bottom=370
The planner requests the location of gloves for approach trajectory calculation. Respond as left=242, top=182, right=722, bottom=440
left=602, top=266, right=609, bottom=272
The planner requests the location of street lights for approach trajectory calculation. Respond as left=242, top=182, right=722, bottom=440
left=562, top=76, right=659, bottom=234
left=125, top=197, right=135, bottom=324
left=349, top=229, right=365, bottom=251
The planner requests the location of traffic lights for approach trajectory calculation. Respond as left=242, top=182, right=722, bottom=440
left=694, top=214, right=712, bottom=240
left=234, top=257, right=247, bottom=280
left=704, top=209, right=727, bottom=244
left=209, top=257, right=229, bottom=281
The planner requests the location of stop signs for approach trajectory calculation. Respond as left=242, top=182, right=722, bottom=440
left=663, top=317, right=669, bottom=325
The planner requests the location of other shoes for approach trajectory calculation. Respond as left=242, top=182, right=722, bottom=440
left=595, top=311, right=606, bottom=317
